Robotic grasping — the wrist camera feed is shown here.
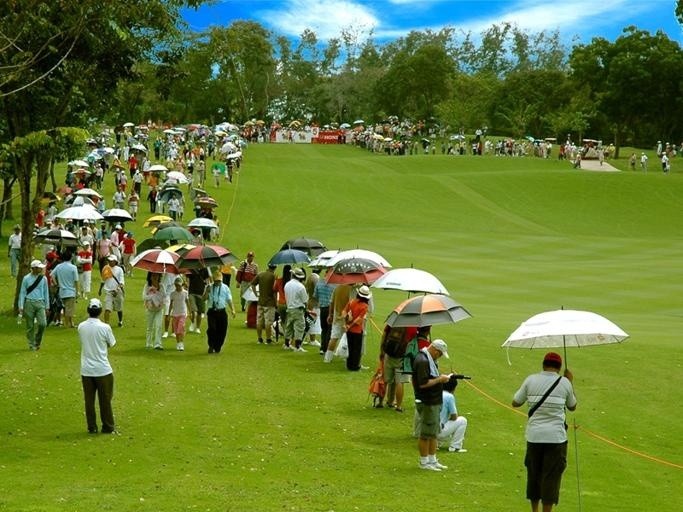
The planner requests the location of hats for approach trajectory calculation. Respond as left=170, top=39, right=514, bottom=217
left=128, top=231, right=134, bottom=236
left=290, top=268, right=305, bottom=278
left=46, top=252, right=59, bottom=258
left=108, top=255, right=117, bottom=262
left=213, top=272, right=221, bottom=281
left=88, top=298, right=102, bottom=309
left=357, top=286, right=371, bottom=299
left=30, top=260, right=46, bottom=268
left=247, top=252, right=254, bottom=257
left=82, top=241, right=90, bottom=246
left=174, top=278, right=182, bottom=285
left=115, top=225, right=121, bottom=229
left=432, top=339, right=449, bottom=359
left=544, top=353, right=562, bottom=365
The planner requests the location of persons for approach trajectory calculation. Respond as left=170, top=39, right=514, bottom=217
left=558, top=135, right=617, bottom=167
left=162, top=274, right=188, bottom=339
left=413, top=339, right=454, bottom=472
left=147, top=181, right=224, bottom=246
left=51, top=249, right=80, bottom=328
left=247, top=301, right=257, bottom=328
left=236, top=252, right=259, bottom=312
left=273, top=265, right=292, bottom=332
left=512, top=351, right=577, bottom=511
left=340, top=286, right=372, bottom=371
left=313, top=268, right=336, bottom=355
left=438, top=376, right=468, bottom=454
left=98, top=254, right=124, bottom=327
left=283, top=268, right=309, bottom=353
left=18, top=259, right=50, bottom=351
left=656, top=141, right=682, bottom=172
left=45, top=252, right=59, bottom=285
left=7, top=224, right=23, bottom=276
left=166, top=275, right=192, bottom=351
left=640, top=152, right=648, bottom=173
left=78, top=298, right=120, bottom=433
left=202, top=270, right=235, bottom=353
left=403, top=325, right=432, bottom=381
left=143, top=271, right=166, bottom=350
left=349, top=283, right=375, bottom=370
left=79, top=240, right=93, bottom=299
left=251, top=262, right=278, bottom=346
left=630, top=152, right=636, bottom=171
left=323, top=285, right=352, bottom=364
left=305, top=265, right=322, bottom=347
left=240, top=125, right=349, bottom=145
left=154, top=126, right=245, bottom=188
left=33, top=185, right=140, bottom=259
left=220, top=264, right=237, bottom=288
left=61, top=122, right=151, bottom=197
left=170, top=239, right=178, bottom=246
left=352, top=112, right=552, bottom=159
left=379, top=324, right=417, bottom=413
left=187, top=267, right=211, bottom=334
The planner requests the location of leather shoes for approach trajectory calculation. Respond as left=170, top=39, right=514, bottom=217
left=208, top=346, right=213, bottom=353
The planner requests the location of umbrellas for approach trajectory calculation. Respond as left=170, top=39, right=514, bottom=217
left=54, top=205, right=103, bottom=231
left=219, top=139, right=244, bottom=160
left=143, top=215, right=196, bottom=241
left=325, top=256, right=387, bottom=285
left=67, top=148, right=101, bottom=167
left=86, top=132, right=105, bottom=160
left=370, top=262, right=450, bottom=300
left=187, top=217, right=218, bottom=228
left=162, top=122, right=212, bottom=138
left=135, top=239, right=169, bottom=254
left=129, top=248, right=181, bottom=273
left=364, top=130, right=393, bottom=142
left=383, top=293, right=474, bottom=341
left=45, top=285, right=64, bottom=327
left=164, top=244, right=197, bottom=256
left=155, top=184, right=182, bottom=200
left=190, top=187, right=217, bottom=209
left=216, top=122, right=238, bottom=143
left=500, top=305, right=628, bottom=371
left=279, top=236, right=328, bottom=258
left=99, top=146, right=115, bottom=156
left=153, top=226, right=196, bottom=241
left=101, top=208, right=133, bottom=223
left=175, top=242, right=240, bottom=268
left=72, top=188, right=101, bottom=199
left=266, top=244, right=312, bottom=268
left=150, top=164, right=188, bottom=185
left=123, top=122, right=149, bottom=154
left=300, top=310, right=316, bottom=346
left=367, top=362, right=386, bottom=407
left=306, top=247, right=343, bottom=269
left=244, top=118, right=365, bottom=128
left=36, top=228, right=78, bottom=252
left=240, top=284, right=261, bottom=302
left=322, top=245, right=393, bottom=268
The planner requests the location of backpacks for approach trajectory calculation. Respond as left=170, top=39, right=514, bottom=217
left=403, top=338, right=418, bottom=371
left=382, top=327, right=406, bottom=358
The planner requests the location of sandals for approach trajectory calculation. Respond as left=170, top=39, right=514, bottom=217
left=396, top=408, right=405, bottom=413
left=388, top=403, right=396, bottom=408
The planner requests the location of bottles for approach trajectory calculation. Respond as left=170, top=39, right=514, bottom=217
left=16, top=311, right=23, bottom=325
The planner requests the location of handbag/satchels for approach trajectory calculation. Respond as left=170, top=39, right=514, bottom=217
left=236, top=261, right=247, bottom=283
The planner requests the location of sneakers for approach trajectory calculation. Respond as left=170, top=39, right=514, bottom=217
left=265, top=339, right=277, bottom=345
left=436, top=441, right=440, bottom=450
left=177, top=343, right=180, bottom=350
left=360, top=365, right=370, bottom=370
left=319, top=350, right=324, bottom=355
left=449, top=447, right=467, bottom=452
left=420, top=463, right=441, bottom=472
left=324, top=357, right=332, bottom=363
left=189, top=322, right=194, bottom=332
left=171, top=333, right=175, bottom=336
left=256, top=338, right=265, bottom=344
left=162, top=332, right=168, bottom=337
left=89, top=429, right=97, bottom=432
left=284, top=345, right=293, bottom=350
left=294, top=348, right=307, bottom=353
left=118, top=321, right=122, bottom=326
left=309, top=340, right=320, bottom=346
left=101, top=429, right=116, bottom=434
left=430, top=463, right=448, bottom=469
left=155, top=345, right=163, bottom=350
left=196, top=328, right=200, bottom=334
left=146, top=344, right=153, bottom=348
left=180, top=344, right=185, bottom=351
